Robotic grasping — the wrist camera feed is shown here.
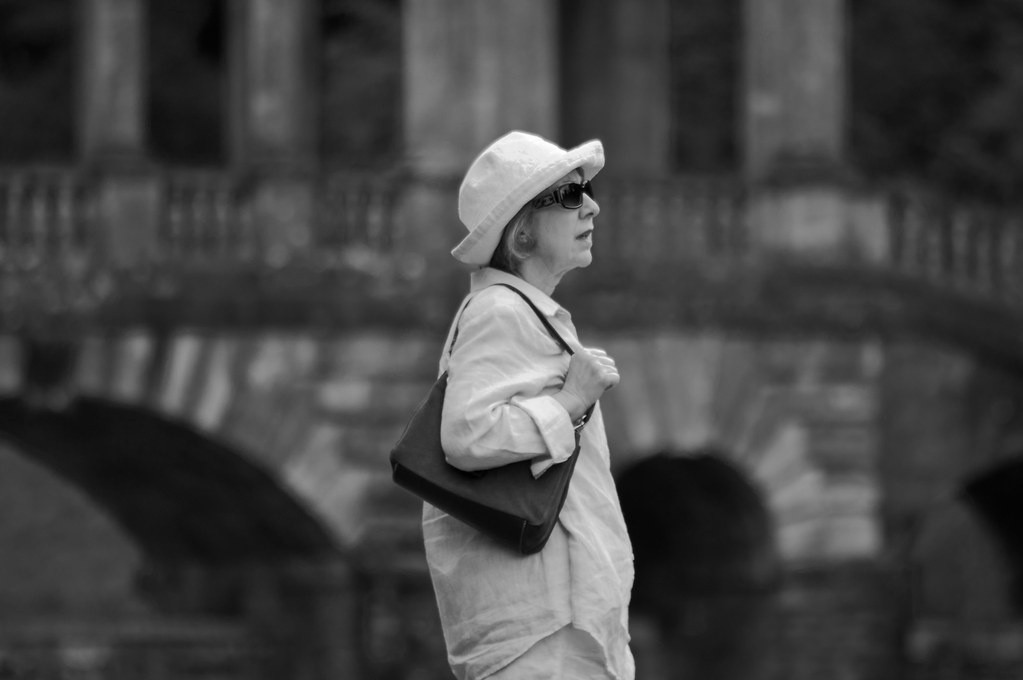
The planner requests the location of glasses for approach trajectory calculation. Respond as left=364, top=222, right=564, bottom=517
left=537, top=181, right=595, bottom=209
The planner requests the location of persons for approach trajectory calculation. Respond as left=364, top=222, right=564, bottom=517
left=391, top=130, right=636, bottom=679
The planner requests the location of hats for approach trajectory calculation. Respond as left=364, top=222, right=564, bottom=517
left=450, top=129, right=606, bottom=267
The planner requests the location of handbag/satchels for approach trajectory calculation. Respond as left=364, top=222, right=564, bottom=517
left=390, top=283, right=597, bottom=555
